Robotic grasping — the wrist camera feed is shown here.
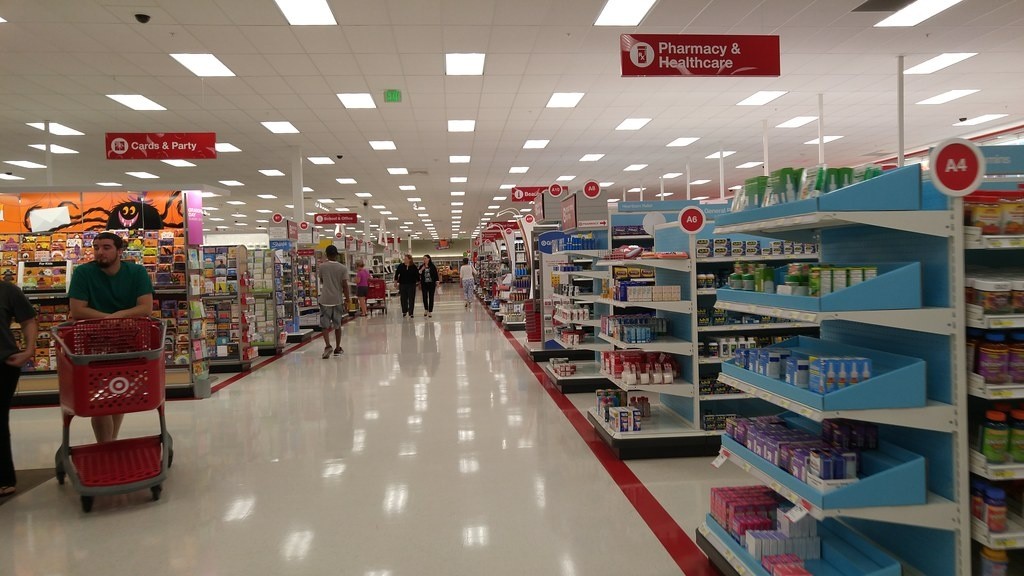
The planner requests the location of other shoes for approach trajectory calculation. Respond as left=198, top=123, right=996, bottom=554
left=424, top=312, right=427, bottom=316
left=429, top=314, right=432, bottom=317
left=333, top=346, right=344, bottom=356
left=321, top=345, right=333, bottom=359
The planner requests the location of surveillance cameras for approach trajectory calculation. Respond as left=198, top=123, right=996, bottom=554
left=135, top=14, right=150, bottom=24
left=338, top=155, right=343, bottom=159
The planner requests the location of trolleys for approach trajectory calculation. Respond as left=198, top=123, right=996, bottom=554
left=51, top=313, right=173, bottom=513
left=365, top=278, right=388, bottom=317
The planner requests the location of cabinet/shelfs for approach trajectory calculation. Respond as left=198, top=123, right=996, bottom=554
left=268, top=238, right=315, bottom=343
left=523, top=225, right=595, bottom=362
left=338, top=249, right=400, bottom=325
left=249, top=248, right=292, bottom=355
left=0, top=189, right=219, bottom=407
left=589, top=206, right=819, bottom=462
left=202, top=244, right=261, bottom=374
left=496, top=207, right=561, bottom=331
left=688, top=135, right=1024, bottom=576
left=469, top=238, right=506, bottom=315
left=485, top=223, right=527, bottom=321
left=297, top=248, right=334, bottom=331
left=545, top=229, right=644, bottom=395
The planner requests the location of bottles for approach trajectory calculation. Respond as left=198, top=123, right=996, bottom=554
left=970, top=399, right=1024, bottom=465
left=970, top=477, right=1008, bottom=534
left=965, top=329, right=1024, bottom=385
left=965, top=271, right=1024, bottom=314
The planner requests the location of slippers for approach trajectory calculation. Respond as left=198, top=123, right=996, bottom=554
left=0, top=486, right=16, bottom=497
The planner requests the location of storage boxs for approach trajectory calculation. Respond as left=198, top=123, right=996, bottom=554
left=0, top=228, right=288, bottom=372
left=549, top=164, right=928, bottom=576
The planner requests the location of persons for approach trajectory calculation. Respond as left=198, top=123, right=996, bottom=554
left=394, top=254, right=421, bottom=318
left=68, top=232, right=156, bottom=442
left=0, top=280, right=37, bottom=497
left=396, top=318, right=441, bottom=378
left=318, top=245, right=350, bottom=358
left=354, top=261, right=373, bottom=315
left=418, top=255, right=440, bottom=317
left=459, top=258, right=478, bottom=310
